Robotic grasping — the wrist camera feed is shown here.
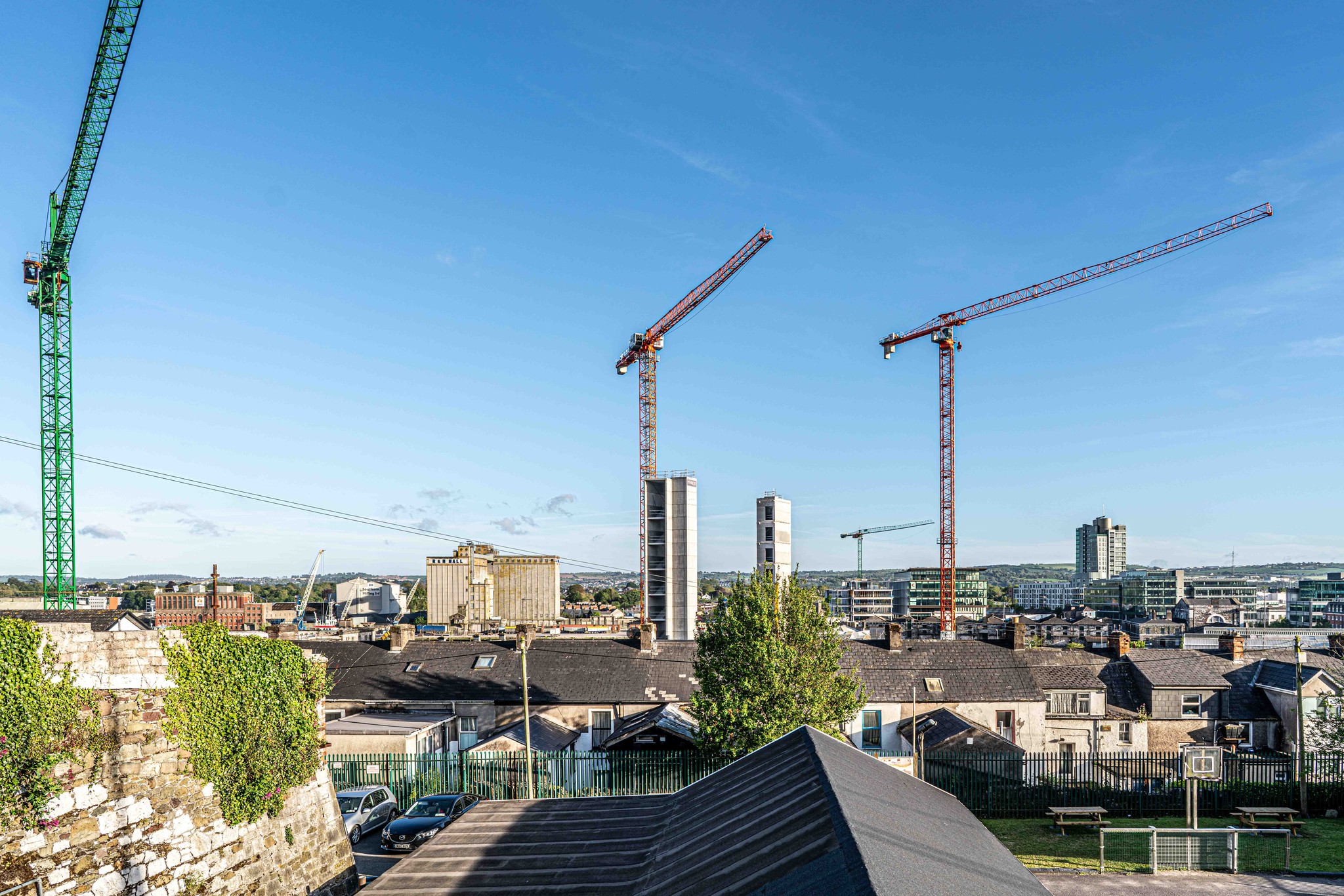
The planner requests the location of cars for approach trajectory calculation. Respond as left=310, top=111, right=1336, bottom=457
left=381, top=791, right=489, bottom=852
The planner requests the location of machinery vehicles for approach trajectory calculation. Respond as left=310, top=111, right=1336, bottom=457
left=292, top=549, right=326, bottom=630
left=340, top=576, right=361, bottom=621
left=385, top=578, right=421, bottom=630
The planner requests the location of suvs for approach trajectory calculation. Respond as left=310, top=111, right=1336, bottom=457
left=334, top=785, right=399, bottom=844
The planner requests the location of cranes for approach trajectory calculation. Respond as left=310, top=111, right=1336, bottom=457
left=840, top=520, right=938, bottom=577
left=21, top=0, right=145, bottom=611
left=613, top=221, right=772, bottom=625
left=878, top=202, right=1273, bottom=640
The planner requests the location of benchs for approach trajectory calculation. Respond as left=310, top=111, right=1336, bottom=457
left=1248, top=821, right=1307, bottom=824
left=1055, top=821, right=1112, bottom=825
left=1045, top=813, right=1098, bottom=816
left=1229, top=813, right=1285, bottom=816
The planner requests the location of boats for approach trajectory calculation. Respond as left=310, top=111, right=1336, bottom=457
left=314, top=593, right=339, bottom=631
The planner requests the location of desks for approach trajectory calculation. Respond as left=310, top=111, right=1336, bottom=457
left=1048, top=806, right=1108, bottom=835
left=1234, top=807, right=1299, bottom=835
